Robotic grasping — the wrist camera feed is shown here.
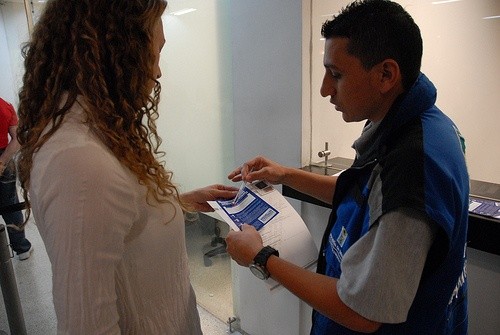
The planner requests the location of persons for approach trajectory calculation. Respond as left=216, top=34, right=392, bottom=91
left=17, top=0, right=240, bottom=334
left=224, top=0, right=471, bottom=335
left=0, top=95, right=33, bottom=260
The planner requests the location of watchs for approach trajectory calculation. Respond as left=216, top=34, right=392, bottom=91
left=249, top=244, right=279, bottom=281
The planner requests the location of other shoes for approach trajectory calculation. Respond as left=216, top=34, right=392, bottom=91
left=18, top=243, right=32, bottom=260
